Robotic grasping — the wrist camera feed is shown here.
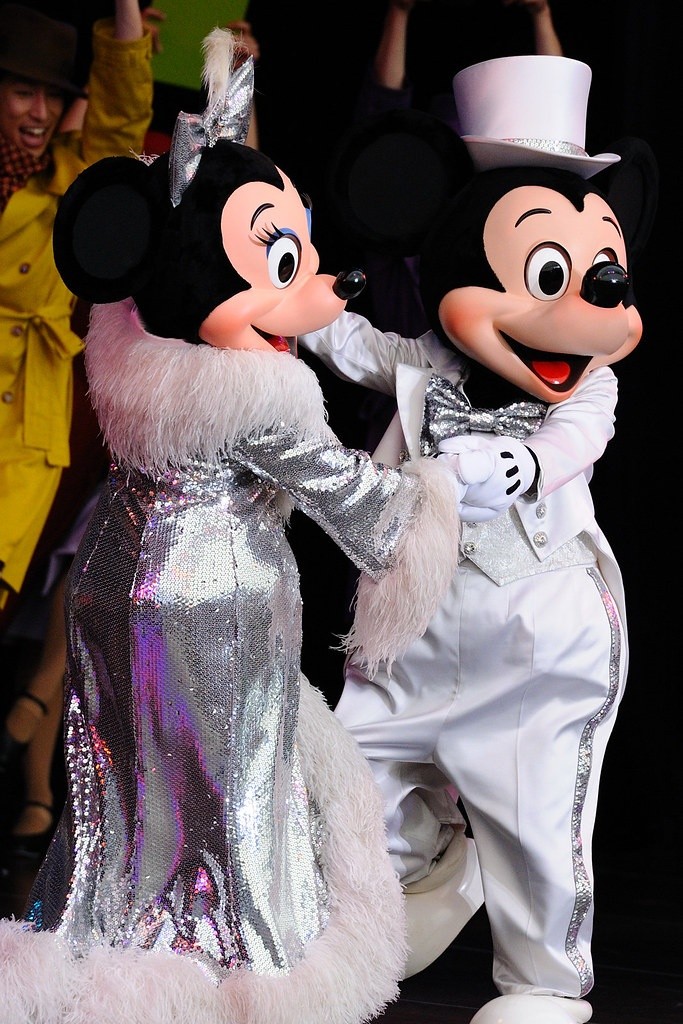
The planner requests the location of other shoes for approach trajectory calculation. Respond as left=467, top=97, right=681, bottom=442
left=0, top=691, right=48, bottom=757
left=9, top=798, right=57, bottom=859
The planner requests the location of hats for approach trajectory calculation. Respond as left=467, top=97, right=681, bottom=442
left=0, top=8, right=91, bottom=100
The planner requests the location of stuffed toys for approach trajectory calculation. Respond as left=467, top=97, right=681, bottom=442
left=4, top=25, right=645, bottom=1024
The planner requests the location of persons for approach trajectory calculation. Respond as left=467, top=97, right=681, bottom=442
left=0, top=0, right=568, bottom=869
